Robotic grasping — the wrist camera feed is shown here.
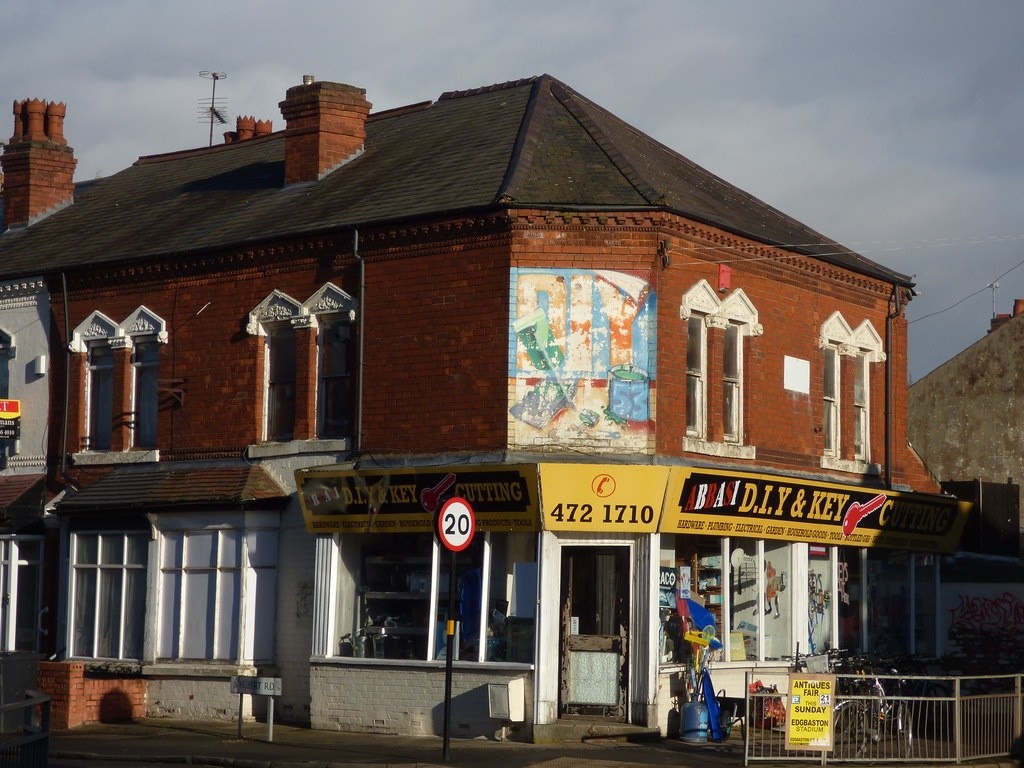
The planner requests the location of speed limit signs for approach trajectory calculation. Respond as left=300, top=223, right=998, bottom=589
left=438, top=497, right=476, bottom=551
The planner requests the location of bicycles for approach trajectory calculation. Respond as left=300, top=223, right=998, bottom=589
left=798, top=649, right=914, bottom=768
